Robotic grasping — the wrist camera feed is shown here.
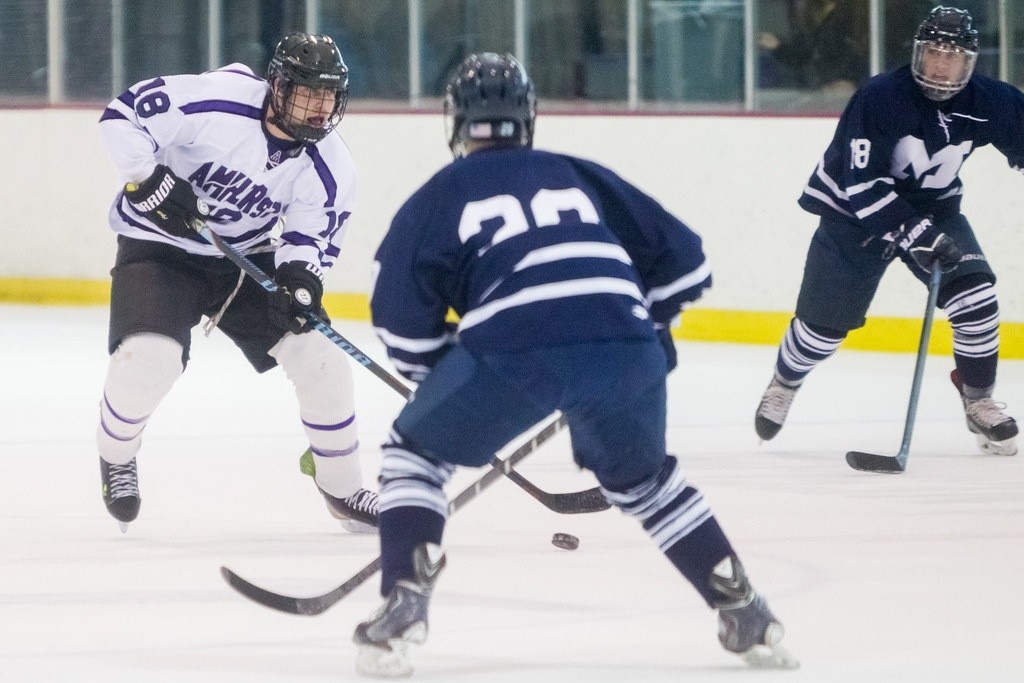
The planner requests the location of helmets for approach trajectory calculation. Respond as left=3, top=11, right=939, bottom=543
left=910, top=6, right=979, bottom=101
left=267, top=30, right=349, bottom=145
left=444, top=52, right=537, bottom=156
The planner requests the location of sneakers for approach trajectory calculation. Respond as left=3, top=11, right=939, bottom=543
left=755, top=376, right=802, bottom=445
left=301, top=448, right=380, bottom=534
left=353, top=542, right=446, bottom=677
left=100, top=456, right=141, bottom=532
left=951, top=368, right=1018, bottom=455
left=707, top=554, right=800, bottom=669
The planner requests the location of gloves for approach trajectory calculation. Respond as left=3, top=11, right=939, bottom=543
left=883, top=216, right=963, bottom=275
left=124, top=164, right=209, bottom=237
left=265, top=260, right=331, bottom=335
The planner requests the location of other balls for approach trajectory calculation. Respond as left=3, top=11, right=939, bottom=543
left=552, top=532, right=581, bottom=551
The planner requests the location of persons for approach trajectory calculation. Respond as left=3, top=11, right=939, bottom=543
left=94, top=33, right=379, bottom=528
left=353, top=52, right=801, bottom=675
left=755, top=5, right=1024, bottom=456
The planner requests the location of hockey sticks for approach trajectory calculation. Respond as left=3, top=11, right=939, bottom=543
left=188, top=218, right=612, bottom=615
left=845, top=260, right=943, bottom=475
